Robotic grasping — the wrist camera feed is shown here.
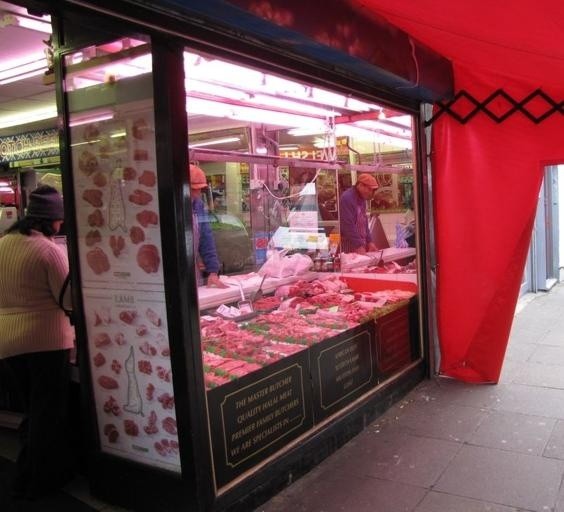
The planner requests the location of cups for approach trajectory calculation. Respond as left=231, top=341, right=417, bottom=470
left=312, top=257, right=333, bottom=272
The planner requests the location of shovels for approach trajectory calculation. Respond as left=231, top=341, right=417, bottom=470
left=256, top=273, right=267, bottom=300
left=238, top=284, right=254, bottom=313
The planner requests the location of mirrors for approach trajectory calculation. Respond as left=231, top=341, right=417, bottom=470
left=333, top=111, right=422, bottom=274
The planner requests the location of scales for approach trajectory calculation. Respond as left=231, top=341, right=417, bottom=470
left=268, top=227, right=328, bottom=249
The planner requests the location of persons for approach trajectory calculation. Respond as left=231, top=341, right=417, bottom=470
left=0, top=184, right=74, bottom=361
left=190, top=164, right=230, bottom=289
left=339, top=173, right=379, bottom=255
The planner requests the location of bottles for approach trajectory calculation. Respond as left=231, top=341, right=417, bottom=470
left=334, top=255, right=341, bottom=272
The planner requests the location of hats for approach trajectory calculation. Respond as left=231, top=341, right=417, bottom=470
left=27, top=185, right=65, bottom=220
left=358, top=173, right=379, bottom=189
left=190, top=163, right=208, bottom=190
